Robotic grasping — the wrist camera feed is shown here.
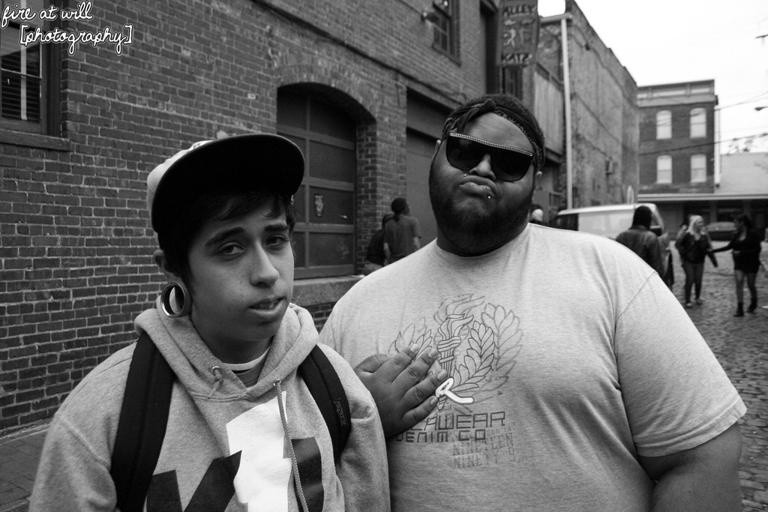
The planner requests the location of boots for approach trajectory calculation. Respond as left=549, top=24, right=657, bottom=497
left=747, top=296, right=758, bottom=312
left=732, top=301, right=745, bottom=317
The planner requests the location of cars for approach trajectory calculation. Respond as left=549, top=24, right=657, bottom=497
left=551, top=201, right=675, bottom=299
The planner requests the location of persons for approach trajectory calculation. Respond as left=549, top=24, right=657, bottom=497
left=706, top=215, right=761, bottom=317
left=28, top=131, right=390, bottom=512
left=364, top=212, right=395, bottom=274
left=382, top=197, right=423, bottom=265
left=617, top=206, right=663, bottom=278
left=674, top=213, right=719, bottom=308
left=317, top=91, right=748, bottom=511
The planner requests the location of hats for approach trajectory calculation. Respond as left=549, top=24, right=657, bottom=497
left=144, top=131, right=305, bottom=234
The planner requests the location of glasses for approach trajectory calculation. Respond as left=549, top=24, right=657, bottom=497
left=442, top=132, right=540, bottom=187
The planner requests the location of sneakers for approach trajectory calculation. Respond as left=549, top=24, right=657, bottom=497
left=695, top=297, right=703, bottom=305
left=685, top=301, right=692, bottom=308
